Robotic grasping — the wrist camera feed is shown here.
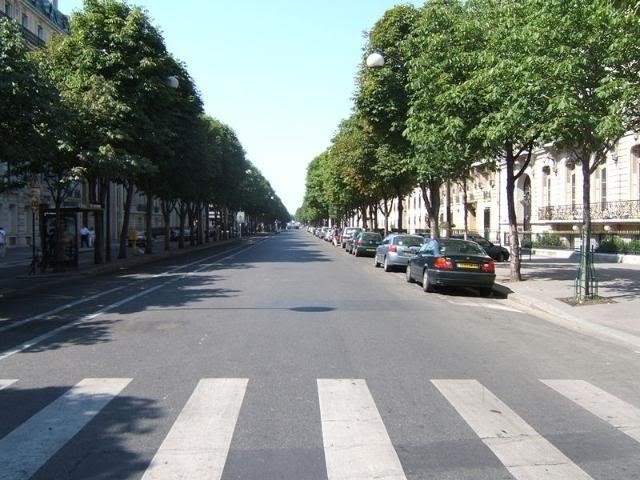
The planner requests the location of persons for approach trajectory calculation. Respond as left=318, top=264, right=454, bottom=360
left=0, top=227, right=9, bottom=254
left=79, top=224, right=89, bottom=248
left=332, top=224, right=339, bottom=248
left=87, top=225, right=95, bottom=249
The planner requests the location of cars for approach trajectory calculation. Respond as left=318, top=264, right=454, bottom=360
left=307, top=225, right=510, bottom=296
left=126, top=231, right=156, bottom=249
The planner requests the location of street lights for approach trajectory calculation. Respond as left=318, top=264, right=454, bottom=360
left=367, top=48, right=452, bottom=239
